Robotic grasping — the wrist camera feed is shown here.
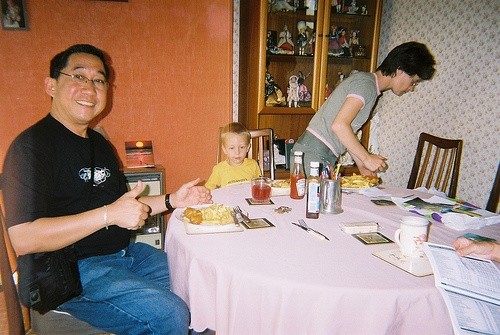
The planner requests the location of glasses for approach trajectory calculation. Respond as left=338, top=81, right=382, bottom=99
left=60, top=71, right=110, bottom=91
left=410, top=75, right=421, bottom=87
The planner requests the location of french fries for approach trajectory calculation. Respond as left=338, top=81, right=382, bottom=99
left=272, top=181, right=290, bottom=188
left=340, top=172, right=373, bottom=188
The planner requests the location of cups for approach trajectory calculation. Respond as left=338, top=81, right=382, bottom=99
left=394, top=216, right=429, bottom=257
left=251, top=177, right=272, bottom=202
left=320, top=179, right=343, bottom=214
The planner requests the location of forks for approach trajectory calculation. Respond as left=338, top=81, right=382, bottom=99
left=233, top=206, right=250, bottom=223
left=298, top=219, right=330, bottom=241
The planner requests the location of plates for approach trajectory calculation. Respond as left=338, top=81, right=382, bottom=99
left=271, top=180, right=290, bottom=189
left=175, top=204, right=248, bottom=226
left=342, top=185, right=378, bottom=192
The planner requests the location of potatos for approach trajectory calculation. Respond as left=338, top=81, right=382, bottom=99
left=184, top=207, right=202, bottom=224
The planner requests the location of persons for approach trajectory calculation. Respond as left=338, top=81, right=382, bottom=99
left=335, top=72, right=345, bottom=86
left=338, top=29, right=349, bottom=55
left=450, top=238, right=500, bottom=262
left=298, top=71, right=311, bottom=101
left=277, top=24, right=294, bottom=54
left=349, top=30, right=360, bottom=57
left=328, top=25, right=344, bottom=56
left=265, top=72, right=286, bottom=106
left=204, top=122, right=262, bottom=190
left=2, top=44, right=208, bottom=335
left=287, top=75, right=299, bottom=108
left=297, top=21, right=307, bottom=55
left=293, top=41, right=436, bottom=178
left=7, top=0, right=21, bottom=24
left=304, top=26, right=313, bottom=54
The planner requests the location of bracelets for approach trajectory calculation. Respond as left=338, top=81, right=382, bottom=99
left=103, top=205, right=108, bottom=229
left=165, top=194, right=173, bottom=210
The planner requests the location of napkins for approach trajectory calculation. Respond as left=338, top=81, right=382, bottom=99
left=357, top=187, right=392, bottom=198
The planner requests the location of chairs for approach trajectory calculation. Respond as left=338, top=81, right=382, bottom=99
left=405, top=132, right=464, bottom=199
left=485, top=159, right=500, bottom=214
left=216, top=126, right=275, bottom=181
left=0, top=171, right=115, bottom=335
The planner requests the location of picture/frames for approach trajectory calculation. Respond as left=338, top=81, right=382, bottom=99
left=265, top=138, right=286, bottom=165
left=285, top=142, right=295, bottom=170
left=257, top=149, right=277, bottom=172
left=0, top=0, right=30, bottom=31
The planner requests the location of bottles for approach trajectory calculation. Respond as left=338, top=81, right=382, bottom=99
left=290, top=151, right=305, bottom=199
left=306, top=162, right=320, bottom=219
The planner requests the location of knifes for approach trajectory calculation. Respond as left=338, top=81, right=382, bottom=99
left=292, top=223, right=325, bottom=240
left=230, top=211, right=240, bottom=227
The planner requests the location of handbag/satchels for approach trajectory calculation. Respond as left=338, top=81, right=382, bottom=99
left=18, top=248, right=82, bottom=314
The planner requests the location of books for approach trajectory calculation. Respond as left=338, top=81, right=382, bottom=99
left=422, top=242, right=500, bottom=335
left=125, top=141, right=155, bottom=168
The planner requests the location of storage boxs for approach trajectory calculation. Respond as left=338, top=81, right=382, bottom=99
left=338, top=220, right=378, bottom=236
left=352, top=44, right=370, bottom=58
left=125, top=139, right=156, bottom=168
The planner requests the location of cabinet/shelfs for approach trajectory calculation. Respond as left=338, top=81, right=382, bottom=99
left=237, top=0, right=383, bottom=182
left=122, top=165, right=165, bottom=250
